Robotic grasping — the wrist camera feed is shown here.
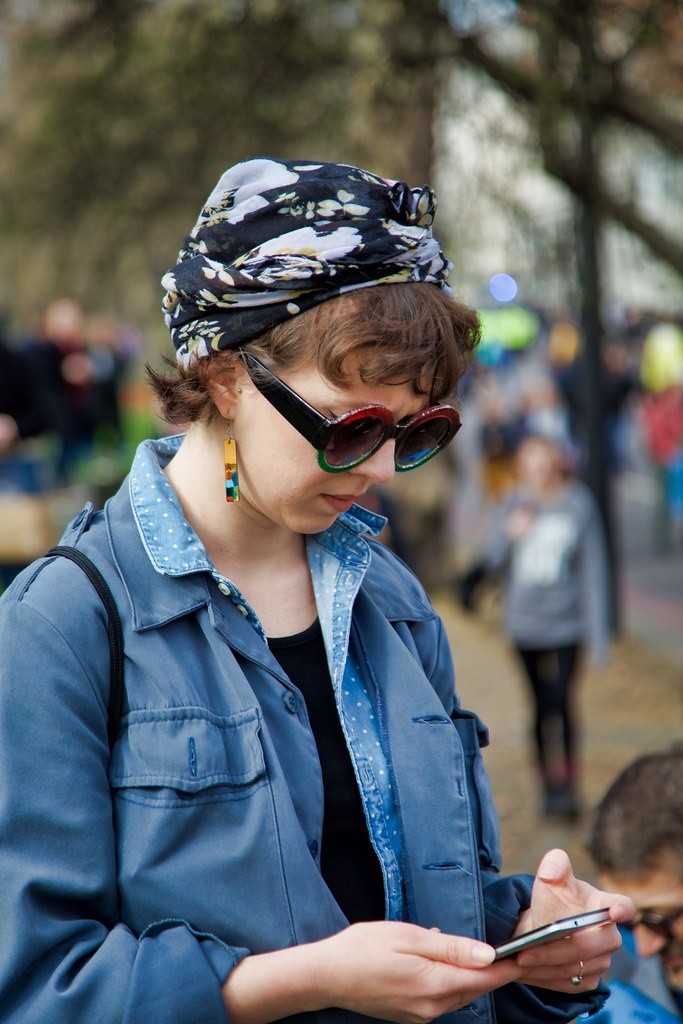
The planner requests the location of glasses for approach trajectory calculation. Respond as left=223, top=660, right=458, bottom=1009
left=237, top=343, right=466, bottom=474
left=619, top=908, right=682, bottom=939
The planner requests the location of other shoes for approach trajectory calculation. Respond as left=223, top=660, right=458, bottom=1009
left=561, top=800, right=578, bottom=821
left=543, top=796, right=559, bottom=813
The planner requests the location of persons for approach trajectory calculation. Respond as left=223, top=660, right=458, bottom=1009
left=0, top=295, right=124, bottom=484
left=584, top=747, right=682, bottom=1024
left=443, top=352, right=683, bottom=824
left=0, top=158, right=637, bottom=1024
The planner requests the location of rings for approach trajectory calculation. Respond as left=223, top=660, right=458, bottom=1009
left=569, top=960, right=584, bottom=985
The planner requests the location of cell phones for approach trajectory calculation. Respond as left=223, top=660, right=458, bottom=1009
left=493, top=906, right=612, bottom=961
